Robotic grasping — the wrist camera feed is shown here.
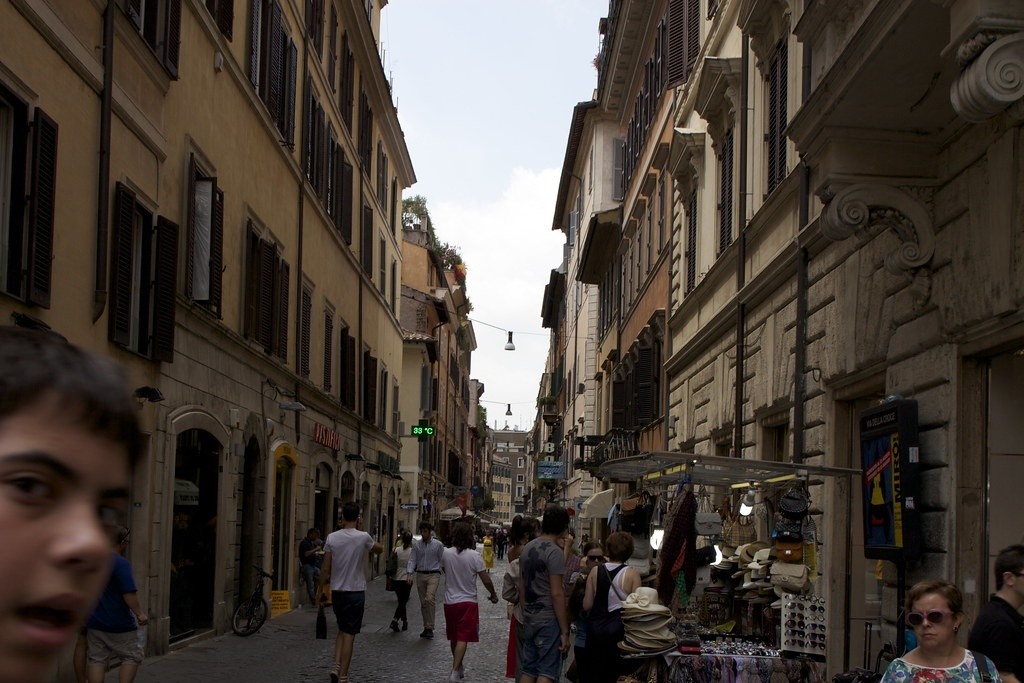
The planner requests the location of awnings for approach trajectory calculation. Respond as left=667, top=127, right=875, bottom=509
left=578, top=489, right=614, bottom=521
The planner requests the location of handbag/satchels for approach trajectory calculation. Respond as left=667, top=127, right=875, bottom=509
left=770, top=562, right=807, bottom=590
left=776, top=542, right=802, bottom=561
left=720, top=511, right=757, bottom=544
left=316, top=608, right=327, bottom=639
left=696, top=546, right=716, bottom=567
left=501, top=573, right=521, bottom=604
left=695, top=513, right=721, bottom=534
left=781, top=494, right=808, bottom=518
left=620, top=492, right=653, bottom=560
left=384, top=554, right=397, bottom=574
left=776, top=520, right=803, bottom=541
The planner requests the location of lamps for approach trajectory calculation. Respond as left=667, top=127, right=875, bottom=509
left=394, top=474, right=405, bottom=481
left=262, top=377, right=306, bottom=419
left=365, top=463, right=380, bottom=471
left=381, top=470, right=393, bottom=477
left=134, top=385, right=164, bottom=409
left=506, top=404, right=512, bottom=415
left=345, top=454, right=366, bottom=463
left=506, top=331, right=515, bottom=351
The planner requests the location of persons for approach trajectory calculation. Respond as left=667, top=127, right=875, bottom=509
left=583, top=531, right=641, bottom=683
left=72, top=524, right=149, bottom=683
left=482, top=530, right=495, bottom=573
left=518, top=505, right=571, bottom=683
left=879, top=576, right=1003, bottom=683
left=567, top=542, right=610, bottom=683
left=298, top=528, right=327, bottom=609
left=314, top=501, right=384, bottom=683
left=389, top=515, right=542, bottom=683
left=967, top=544, right=1024, bottom=683
left=556, top=527, right=582, bottom=581
left=0, top=323, right=150, bottom=683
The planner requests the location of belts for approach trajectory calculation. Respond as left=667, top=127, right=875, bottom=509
left=415, top=570, right=441, bottom=574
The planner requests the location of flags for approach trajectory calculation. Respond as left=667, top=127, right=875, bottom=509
left=455, top=492, right=469, bottom=517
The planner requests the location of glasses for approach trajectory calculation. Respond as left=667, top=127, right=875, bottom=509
left=589, top=556, right=603, bottom=562
left=906, top=610, right=954, bottom=625
left=785, top=594, right=825, bottom=650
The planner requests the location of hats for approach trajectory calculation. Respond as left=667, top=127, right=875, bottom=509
left=714, top=541, right=777, bottom=603
left=617, top=587, right=678, bottom=658
left=770, top=585, right=804, bottom=609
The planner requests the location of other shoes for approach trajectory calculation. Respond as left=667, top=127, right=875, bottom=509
left=311, top=604, right=323, bottom=609
left=420, top=629, right=433, bottom=640
left=390, top=621, right=400, bottom=632
left=329, top=663, right=348, bottom=683
left=299, top=572, right=306, bottom=587
left=450, top=667, right=464, bottom=683
left=402, top=622, right=407, bottom=630
